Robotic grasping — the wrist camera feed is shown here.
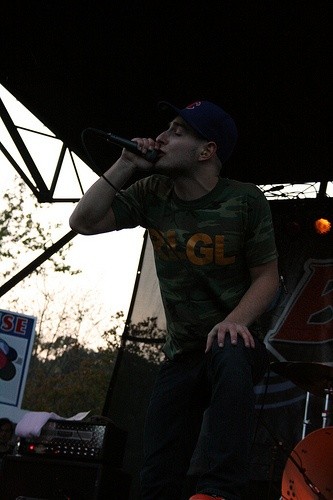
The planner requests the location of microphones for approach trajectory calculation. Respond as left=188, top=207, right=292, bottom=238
left=87, top=127, right=161, bottom=164
left=302, top=473, right=328, bottom=500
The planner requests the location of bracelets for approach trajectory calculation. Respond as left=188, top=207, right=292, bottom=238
left=101, top=174, right=121, bottom=193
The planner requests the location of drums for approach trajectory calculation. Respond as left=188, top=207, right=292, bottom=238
left=279, top=426, right=333, bottom=500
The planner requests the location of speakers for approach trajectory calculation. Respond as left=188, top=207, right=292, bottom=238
left=0, top=456, right=133, bottom=500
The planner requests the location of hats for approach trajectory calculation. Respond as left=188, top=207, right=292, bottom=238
left=156, top=100, right=239, bottom=163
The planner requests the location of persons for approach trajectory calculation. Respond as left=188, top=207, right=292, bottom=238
left=69, top=102, right=280, bottom=500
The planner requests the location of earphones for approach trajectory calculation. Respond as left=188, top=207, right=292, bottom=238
left=200, top=147, right=210, bottom=155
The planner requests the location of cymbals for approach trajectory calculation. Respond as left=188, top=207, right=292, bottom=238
left=281, top=362, right=333, bottom=400
